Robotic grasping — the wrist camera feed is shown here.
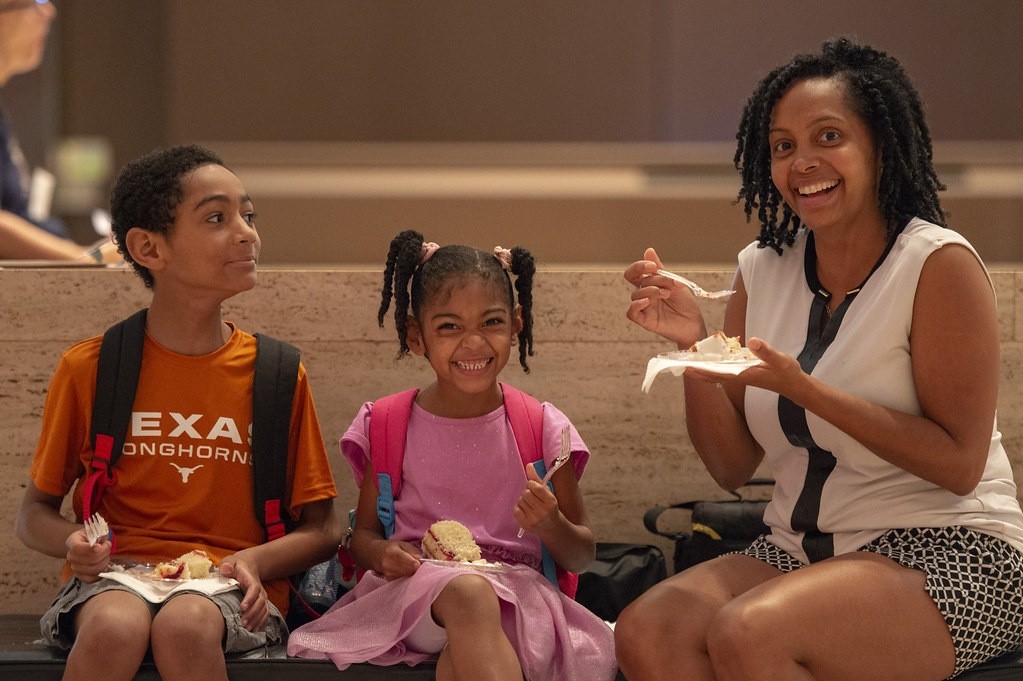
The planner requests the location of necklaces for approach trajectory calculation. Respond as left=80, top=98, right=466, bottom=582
left=802, top=230, right=895, bottom=318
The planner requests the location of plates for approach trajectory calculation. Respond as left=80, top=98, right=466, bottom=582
left=656, top=347, right=762, bottom=363
left=108, top=558, right=223, bottom=590
left=418, top=558, right=510, bottom=574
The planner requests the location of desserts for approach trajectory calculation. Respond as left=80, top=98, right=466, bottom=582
left=688, top=331, right=743, bottom=358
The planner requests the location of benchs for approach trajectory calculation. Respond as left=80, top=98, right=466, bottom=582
left=0, top=265, right=1022, bottom=681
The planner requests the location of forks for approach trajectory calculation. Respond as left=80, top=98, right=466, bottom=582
left=654, top=267, right=737, bottom=298
left=517, top=426, right=572, bottom=538
left=84, top=512, right=110, bottom=547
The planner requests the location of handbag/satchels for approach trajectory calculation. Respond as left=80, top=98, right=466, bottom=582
left=575, top=541, right=667, bottom=623
left=642, top=478, right=775, bottom=573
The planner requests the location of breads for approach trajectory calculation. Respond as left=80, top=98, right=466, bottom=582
left=153, top=548, right=213, bottom=580
left=420, top=517, right=481, bottom=563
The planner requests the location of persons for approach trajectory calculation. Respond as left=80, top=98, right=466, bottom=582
left=287, top=228, right=619, bottom=681
left=0, top=0, right=125, bottom=261
left=15, top=145, right=341, bottom=681
left=613, top=37, right=1023, bottom=681
